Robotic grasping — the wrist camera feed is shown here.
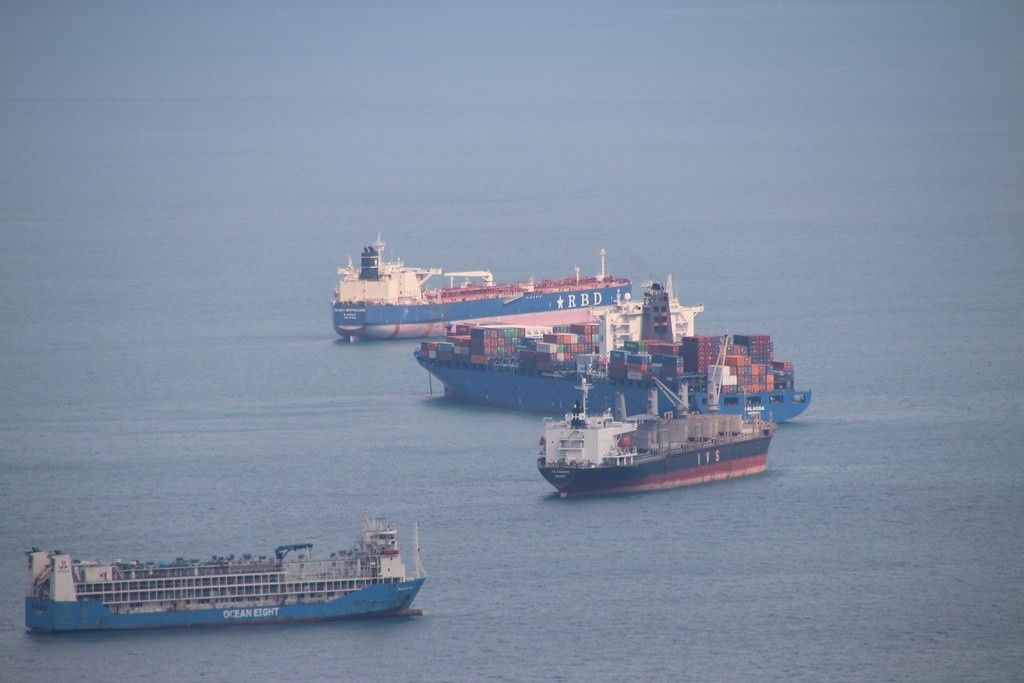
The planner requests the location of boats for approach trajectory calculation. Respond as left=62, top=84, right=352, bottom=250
left=536, top=334, right=778, bottom=499
left=25, top=510, right=428, bottom=635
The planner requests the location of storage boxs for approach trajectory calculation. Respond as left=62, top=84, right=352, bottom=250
left=417, top=321, right=794, bottom=396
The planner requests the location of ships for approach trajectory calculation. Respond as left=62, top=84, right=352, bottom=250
left=331, top=232, right=632, bottom=343
left=413, top=274, right=812, bottom=424
